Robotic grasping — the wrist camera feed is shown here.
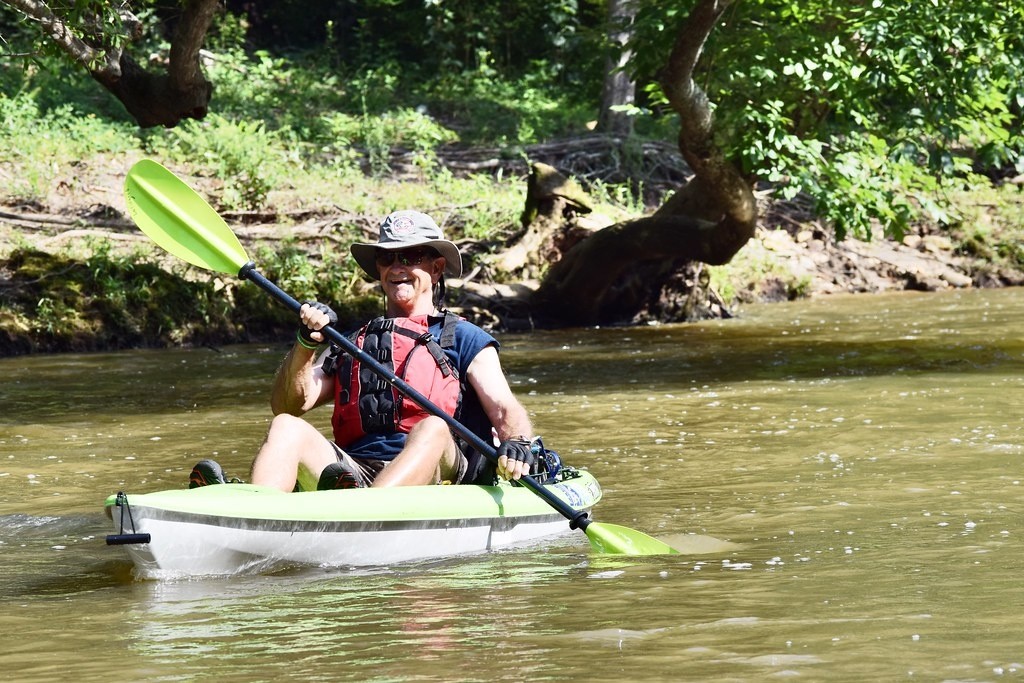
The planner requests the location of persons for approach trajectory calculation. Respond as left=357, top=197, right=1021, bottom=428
left=189, top=210, right=534, bottom=491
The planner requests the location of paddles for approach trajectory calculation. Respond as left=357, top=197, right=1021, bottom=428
left=121, top=157, right=683, bottom=557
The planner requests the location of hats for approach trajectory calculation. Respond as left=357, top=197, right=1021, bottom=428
left=350, top=210, right=462, bottom=281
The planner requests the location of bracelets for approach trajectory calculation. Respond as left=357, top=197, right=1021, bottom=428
left=296, top=328, right=320, bottom=350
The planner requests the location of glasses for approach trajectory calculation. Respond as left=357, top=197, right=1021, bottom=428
left=371, top=249, right=438, bottom=267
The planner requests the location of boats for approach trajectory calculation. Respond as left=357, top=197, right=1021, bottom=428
left=101, top=467, right=604, bottom=580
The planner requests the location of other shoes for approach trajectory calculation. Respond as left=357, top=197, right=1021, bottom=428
left=189, top=459, right=245, bottom=489
left=317, top=463, right=361, bottom=491
